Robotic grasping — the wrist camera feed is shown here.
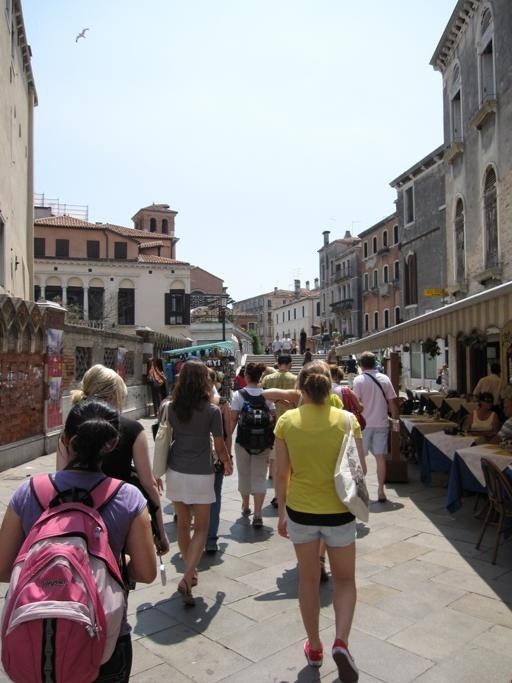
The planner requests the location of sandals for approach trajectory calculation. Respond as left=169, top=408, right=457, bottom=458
left=271, top=497, right=279, bottom=508
left=319, top=557, right=329, bottom=583
left=188, top=565, right=198, bottom=586
left=177, top=579, right=195, bottom=606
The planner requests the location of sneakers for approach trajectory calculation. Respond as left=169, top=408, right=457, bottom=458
left=378, top=486, right=386, bottom=501
left=241, top=508, right=263, bottom=527
left=204, top=541, right=217, bottom=553
left=304, top=636, right=324, bottom=667
left=331, top=639, right=360, bottom=683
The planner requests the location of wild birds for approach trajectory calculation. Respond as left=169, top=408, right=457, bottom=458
left=76, top=28, right=89, bottom=43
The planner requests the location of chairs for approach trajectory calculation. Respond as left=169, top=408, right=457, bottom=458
left=475, top=458, right=512, bottom=563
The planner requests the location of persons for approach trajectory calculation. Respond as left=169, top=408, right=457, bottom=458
left=462, top=393, right=499, bottom=443
left=56, top=364, right=171, bottom=557
left=1, top=397, right=157, bottom=682
left=145, top=346, right=399, bottom=580
left=266, top=326, right=342, bottom=357
left=470, top=359, right=502, bottom=406
left=272, top=358, right=370, bottom=683
left=485, top=395, right=511, bottom=450
left=156, top=359, right=233, bottom=606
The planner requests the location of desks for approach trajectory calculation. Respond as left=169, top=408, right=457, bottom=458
left=400, top=415, right=512, bottom=538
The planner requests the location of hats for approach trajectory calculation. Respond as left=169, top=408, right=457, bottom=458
left=278, top=353, right=291, bottom=364
left=475, top=392, right=495, bottom=403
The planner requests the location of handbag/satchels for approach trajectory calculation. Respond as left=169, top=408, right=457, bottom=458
left=151, top=422, right=160, bottom=443
left=339, top=387, right=366, bottom=431
left=127, top=467, right=162, bottom=552
left=211, top=447, right=232, bottom=473
left=334, top=411, right=370, bottom=524
left=153, top=403, right=174, bottom=478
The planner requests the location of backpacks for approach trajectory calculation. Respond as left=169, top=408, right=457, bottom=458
left=237, top=389, right=274, bottom=453
left=146, top=359, right=167, bottom=387
left=0, top=474, right=131, bottom=682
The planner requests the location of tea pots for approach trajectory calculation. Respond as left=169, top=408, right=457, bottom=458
left=442, top=426, right=464, bottom=435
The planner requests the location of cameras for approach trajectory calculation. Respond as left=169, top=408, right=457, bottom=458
left=160, top=564, right=166, bottom=586
